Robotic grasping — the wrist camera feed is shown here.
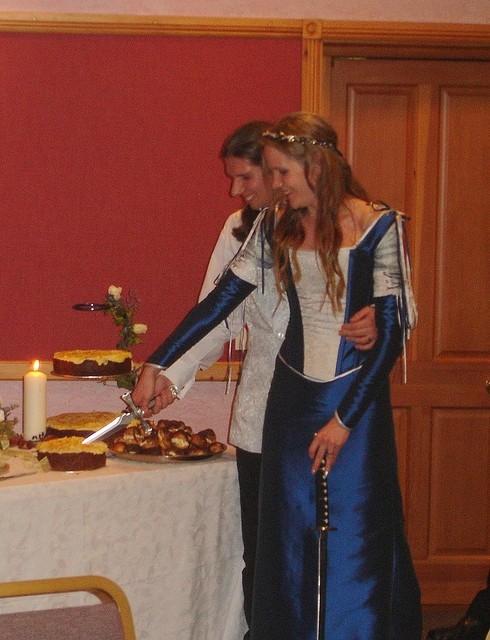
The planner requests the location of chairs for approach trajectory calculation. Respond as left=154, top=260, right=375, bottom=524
left=1, top=574, right=136, bottom=639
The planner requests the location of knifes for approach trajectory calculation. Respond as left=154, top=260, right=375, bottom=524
left=81, top=384, right=178, bottom=446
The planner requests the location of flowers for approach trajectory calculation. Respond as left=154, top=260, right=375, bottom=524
left=102, top=283, right=148, bottom=350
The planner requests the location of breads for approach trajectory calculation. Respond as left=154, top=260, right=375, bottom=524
left=114, top=420, right=225, bottom=455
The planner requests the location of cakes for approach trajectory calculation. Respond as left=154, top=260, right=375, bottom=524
left=36, top=436, right=110, bottom=472
left=53, top=348, right=132, bottom=377
left=46, top=410, right=135, bottom=439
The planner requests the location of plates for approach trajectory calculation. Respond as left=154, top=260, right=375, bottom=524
left=49, top=372, right=141, bottom=383
left=111, top=443, right=231, bottom=463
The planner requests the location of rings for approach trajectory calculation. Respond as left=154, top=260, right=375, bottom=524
left=366, top=335, right=371, bottom=343
left=327, top=452, right=335, bottom=454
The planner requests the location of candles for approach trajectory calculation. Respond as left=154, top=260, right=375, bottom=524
left=23, top=359, right=47, bottom=443
